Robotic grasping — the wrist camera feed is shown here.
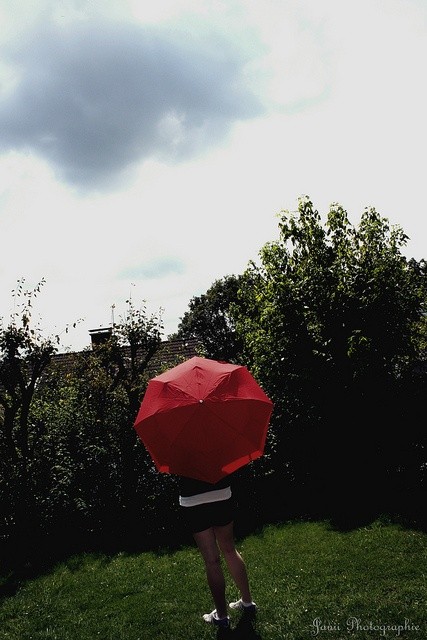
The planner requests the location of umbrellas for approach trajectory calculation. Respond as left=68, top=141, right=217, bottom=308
left=134, top=356, right=274, bottom=486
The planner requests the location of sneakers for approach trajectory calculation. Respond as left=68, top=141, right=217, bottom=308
left=202, top=609, right=231, bottom=627
left=228, top=597, right=256, bottom=613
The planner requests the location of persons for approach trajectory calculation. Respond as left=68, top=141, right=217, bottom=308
left=178, top=478, right=257, bottom=630
